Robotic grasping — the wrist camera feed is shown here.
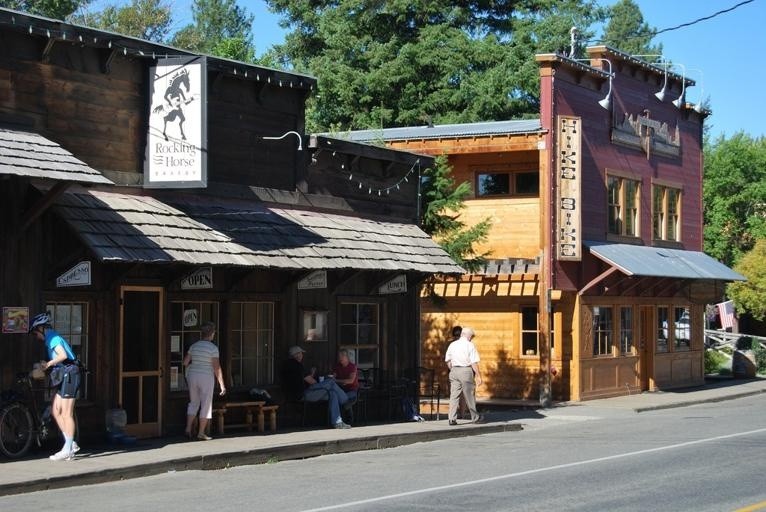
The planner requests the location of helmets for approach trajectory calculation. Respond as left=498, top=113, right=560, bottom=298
left=30, top=313, right=51, bottom=332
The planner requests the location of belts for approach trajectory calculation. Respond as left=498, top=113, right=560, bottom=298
left=453, top=364, right=472, bottom=368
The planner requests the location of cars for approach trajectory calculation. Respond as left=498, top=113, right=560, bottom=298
left=662, top=312, right=691, bottom=343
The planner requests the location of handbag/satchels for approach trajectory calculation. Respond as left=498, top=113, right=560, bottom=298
left=47, top=366, right=65, bottom=389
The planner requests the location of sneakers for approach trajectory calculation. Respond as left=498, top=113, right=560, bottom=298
left=185, top=429, right=193, bottom=440
left=473, top=416, right=489, bottom=424
left=331, top=421, right=351, bottom=429
left=448, top=419, right=460, bottom=425
left=197, top=431, right=213, bottom=440
left=342, top=399, right=357, bottom=411
left=48, top=449, right=75, bottom=461
left=73, top=441, right=81, bottom=453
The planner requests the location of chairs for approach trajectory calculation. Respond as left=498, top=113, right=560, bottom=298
left=274, top=363, right=440, bottom=432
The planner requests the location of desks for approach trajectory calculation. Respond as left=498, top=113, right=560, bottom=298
left=215, top=397, right=266, bottom=436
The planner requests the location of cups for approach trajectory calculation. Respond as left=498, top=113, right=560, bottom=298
left=319, top=376, right=324, bottom=383
left=328, top=375, right=334, bottom=378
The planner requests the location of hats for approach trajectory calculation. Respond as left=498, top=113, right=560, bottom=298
left=287, top=345, right=307, bottom=356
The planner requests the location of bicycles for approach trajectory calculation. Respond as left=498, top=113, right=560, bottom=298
left=0, top=355, right=86, bottom=459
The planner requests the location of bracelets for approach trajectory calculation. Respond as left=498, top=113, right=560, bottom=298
left=47, top=362, right=50, bottom=367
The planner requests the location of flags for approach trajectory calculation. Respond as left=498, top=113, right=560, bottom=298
left=717, top=299, right=736, bottom=330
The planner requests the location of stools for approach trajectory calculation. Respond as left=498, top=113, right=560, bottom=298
left=195, top=407, right=227, bottom=434
left=252, top=405, right=279, bottom=433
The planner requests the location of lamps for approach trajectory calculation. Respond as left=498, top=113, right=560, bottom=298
left=652, top=62, right=684, bottom=109
left=261, top=129, right=303, bottom=152
left=632, top=55, right=668, bottom=101
left=679, top=69, right=703, bottom=114
left=573, top=56, right=612, bottom=112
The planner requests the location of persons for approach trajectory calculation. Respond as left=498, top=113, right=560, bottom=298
left=182, top=322, right=226, bottom=440
left=288, top=347, right=357, bottom=429
left=447, top=326, right=475, bottom=418
left=332, top=349, right=359, bottom=405
left=444, top=327, right=482, bottom=426
left=28, top=313, right=82, bottom=460
